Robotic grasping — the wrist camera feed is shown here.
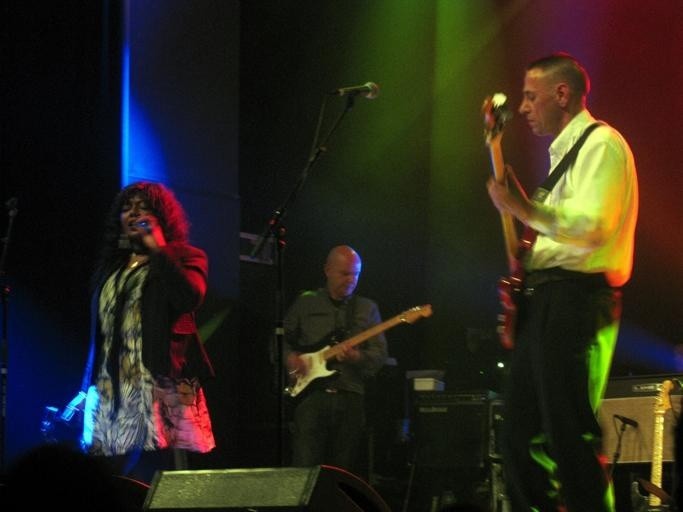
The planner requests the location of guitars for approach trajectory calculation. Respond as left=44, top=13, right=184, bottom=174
left=283, top=304, right=432, bottom=399
left=481, top=93, right=539, bottom=350
left=632, top=381, right=674, bottom=510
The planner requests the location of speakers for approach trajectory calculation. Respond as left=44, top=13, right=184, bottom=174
left=409, top=392, right=490, bottom=471
left=143, top=464, right=323, bottom=511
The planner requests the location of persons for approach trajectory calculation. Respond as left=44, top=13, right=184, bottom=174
left=486, top=53, right=640, bottom=512
left=61, top=182, right=217, bottom=472
left=274, top=243, right=388, bottom=474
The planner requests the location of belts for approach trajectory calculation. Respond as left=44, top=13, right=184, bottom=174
left=521, top=269, right=577, bottom=287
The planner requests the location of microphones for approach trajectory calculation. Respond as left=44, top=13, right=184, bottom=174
left=617, top=413, right=638, bottom=428
left=128, top=220, right=147, bottom=228
left=338, top=81, right=380, bottom=99
